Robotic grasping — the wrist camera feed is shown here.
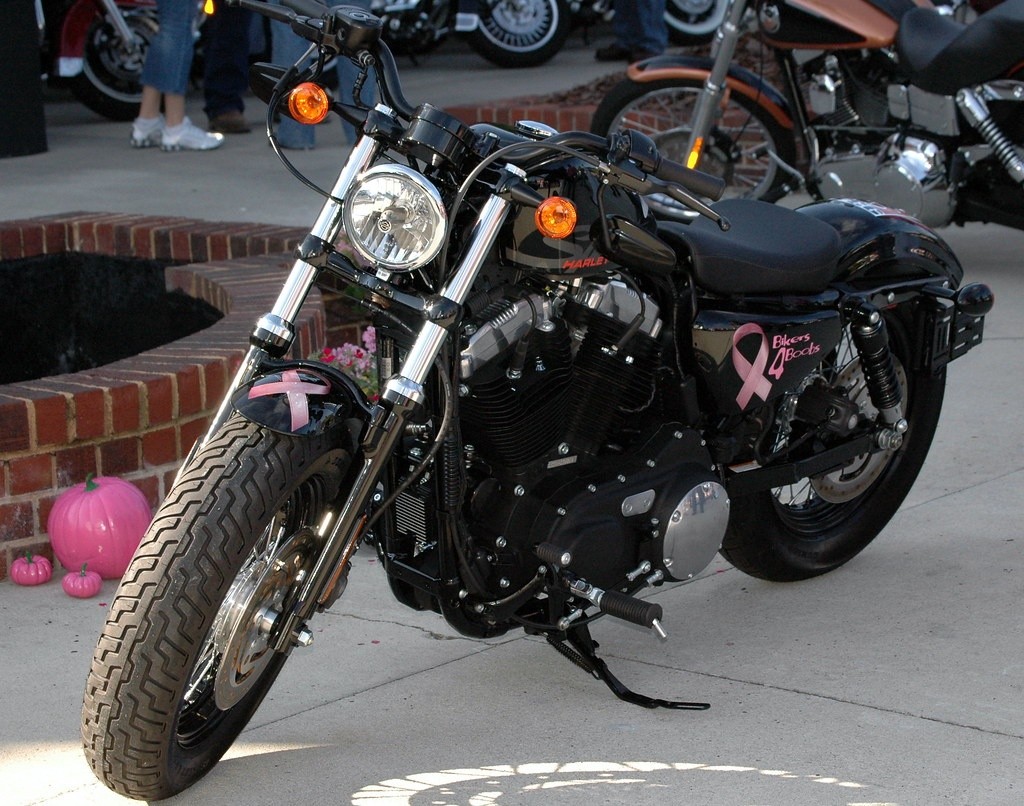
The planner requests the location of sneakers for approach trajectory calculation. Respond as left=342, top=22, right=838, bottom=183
left=128, top=116, right=163, bottom=148
left=205, top=110, right=251, bottom=134
left=161, top=116, right=227, bottom=153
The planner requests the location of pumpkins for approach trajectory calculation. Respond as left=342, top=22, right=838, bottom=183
left=46, top=471, right=153, bottom=580
left=10, top=551, right=52, bottom=586
left=61, top=562, right=102, bottom=598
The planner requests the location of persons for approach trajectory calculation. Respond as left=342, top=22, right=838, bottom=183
left=130, top=0, right=224, bottom=152
left=270, top=0, right=378, bottom=147
left=203, top=1, right=333, bottom=133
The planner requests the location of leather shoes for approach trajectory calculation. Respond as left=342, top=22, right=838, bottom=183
left=594, top=44, right=629, bottom=60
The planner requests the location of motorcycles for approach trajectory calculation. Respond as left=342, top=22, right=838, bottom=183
left=31, top=0, right=730, bottom=132
left=591, top=0, right=1023, bottom=230
left=77, top=2, right=996, bottom=802
